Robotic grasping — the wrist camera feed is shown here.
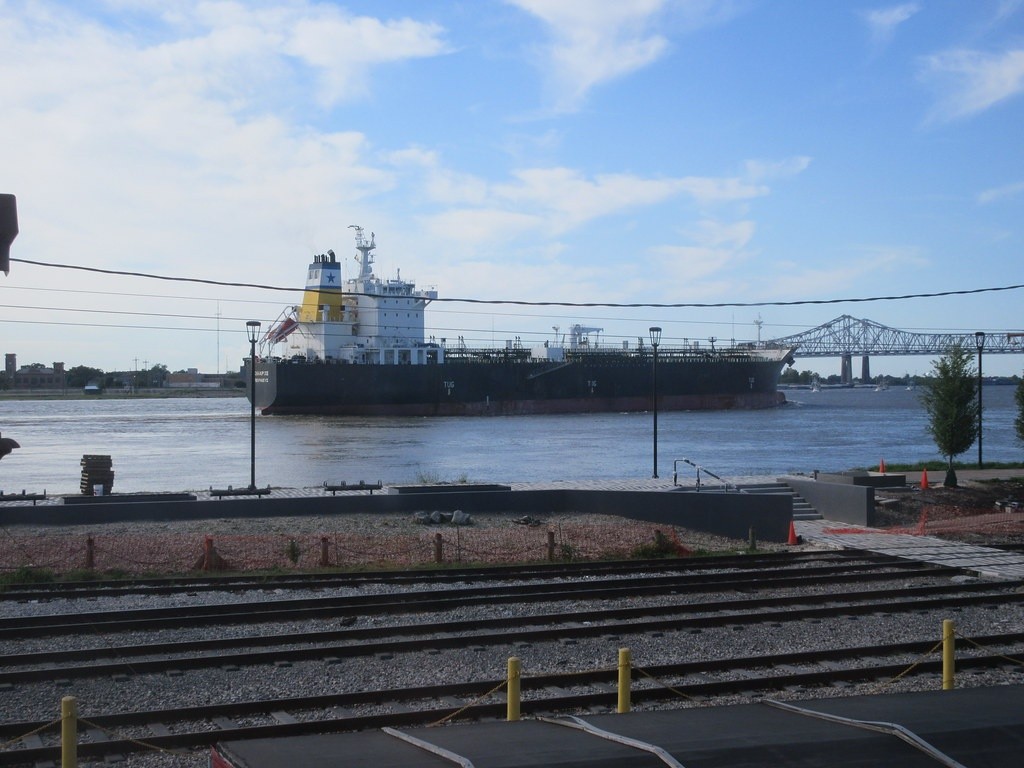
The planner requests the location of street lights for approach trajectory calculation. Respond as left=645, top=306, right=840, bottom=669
left=649, top=328, right=661, bottom=478
left=246, top=321, right=261, bottom=489
left=975, top=332, right=985, bottom=469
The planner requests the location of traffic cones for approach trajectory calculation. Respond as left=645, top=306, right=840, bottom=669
left=880, top=459, right=884, bottom=473
left=921, top=469, right=928, bottom=488
left=788, top=520, right=797, bottom=545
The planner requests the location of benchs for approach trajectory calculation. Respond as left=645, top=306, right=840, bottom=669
left=0, top=488, right=46, bottom=505
left=321, top=478, right=386, bottom=495
left=208, top=484, right=271, bottom=499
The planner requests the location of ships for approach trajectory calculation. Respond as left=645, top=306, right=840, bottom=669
left=243, top=225, right=798, bottom=417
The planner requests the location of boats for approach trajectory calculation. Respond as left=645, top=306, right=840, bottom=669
left=905, top=387, right=911, bottom=391
left=810, top=377, right=821, bottom=392
left=873, top=387, right=881, bottom=391
